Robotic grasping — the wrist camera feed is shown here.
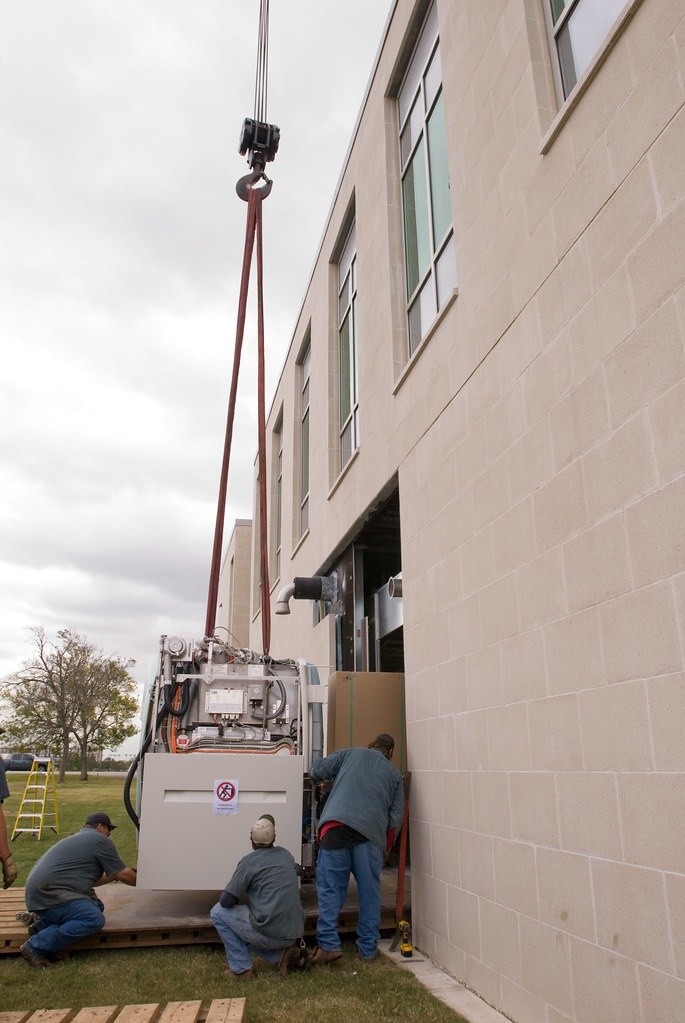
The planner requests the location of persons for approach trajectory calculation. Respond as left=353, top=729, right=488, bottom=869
left=16, top=812, right=137, bottom=969
left=310, top=734, right=405, bottom=965
left=0, top=727, right=18, bottom=889
left=210, top=814, right=305, bottom=981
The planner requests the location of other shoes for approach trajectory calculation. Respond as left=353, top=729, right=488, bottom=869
left=28, top=924, right=39, bottom=936
left=20, top=944, right=44, bottom=967
left=277, top=948, right=298, bottom=978
left=224, top=969, right=256, bottom=978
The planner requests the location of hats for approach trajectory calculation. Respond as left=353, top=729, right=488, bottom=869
left=86, top=812, right=118, bottom=830
left=251, top=814, right=276, bottom=847
left=377, top=733, right=395, bottom=747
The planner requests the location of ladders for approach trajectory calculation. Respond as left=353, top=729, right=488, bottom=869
left=10, top=757, right=60, bottom=841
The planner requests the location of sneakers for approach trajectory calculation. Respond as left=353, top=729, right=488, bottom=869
left=310, top=946, right=344, bottom=964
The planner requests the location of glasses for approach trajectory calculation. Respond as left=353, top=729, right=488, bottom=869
left=102, top=823, right=113, bottom=831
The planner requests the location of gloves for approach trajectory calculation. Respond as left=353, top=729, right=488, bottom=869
left=2, top=853, right=18, bottom=890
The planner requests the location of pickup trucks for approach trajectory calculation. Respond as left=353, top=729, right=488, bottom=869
left=0, top=753, right=51, bottom=772
